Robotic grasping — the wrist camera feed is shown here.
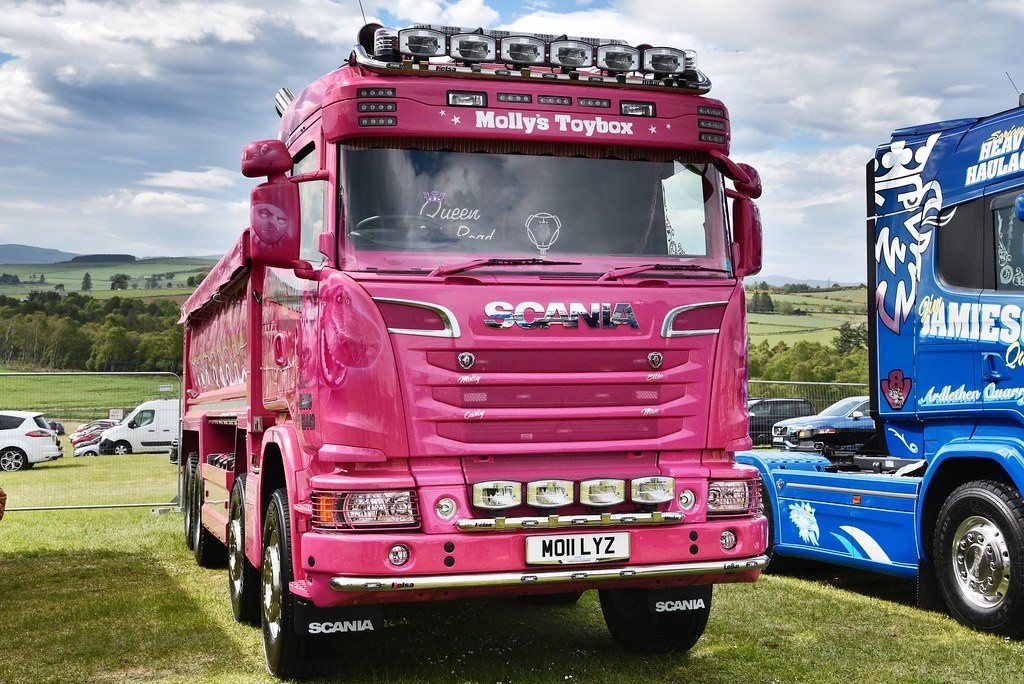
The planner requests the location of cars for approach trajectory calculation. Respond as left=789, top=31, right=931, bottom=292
left=772, top=396, right=875, bottom=452
left=0, top=409, right=64, bottom=472
left=69, top=420, right=121, bottom=457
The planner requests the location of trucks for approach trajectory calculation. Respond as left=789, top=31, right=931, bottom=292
left=169, top=19, right=770, bottom=682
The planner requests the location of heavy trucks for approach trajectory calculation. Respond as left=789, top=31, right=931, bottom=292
left=729, top=94, right=1024, bottom=637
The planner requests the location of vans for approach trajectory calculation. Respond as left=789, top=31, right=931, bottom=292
left=99, top=398, right=182, bottom=455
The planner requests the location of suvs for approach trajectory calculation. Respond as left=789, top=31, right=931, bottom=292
left=746, top=396, right=818, bottom=446
